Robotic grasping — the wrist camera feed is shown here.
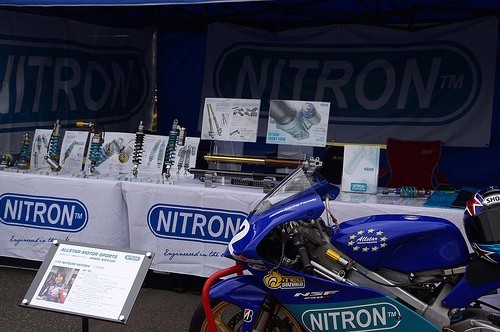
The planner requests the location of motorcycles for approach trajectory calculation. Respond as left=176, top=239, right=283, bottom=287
left=188, top=165, right=500, bottom=332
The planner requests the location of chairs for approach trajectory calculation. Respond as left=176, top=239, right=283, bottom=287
left=374, top=139, right=450, bottom=195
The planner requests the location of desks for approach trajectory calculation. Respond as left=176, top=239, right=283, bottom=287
left=0, top=166, right=474, bottom=294
